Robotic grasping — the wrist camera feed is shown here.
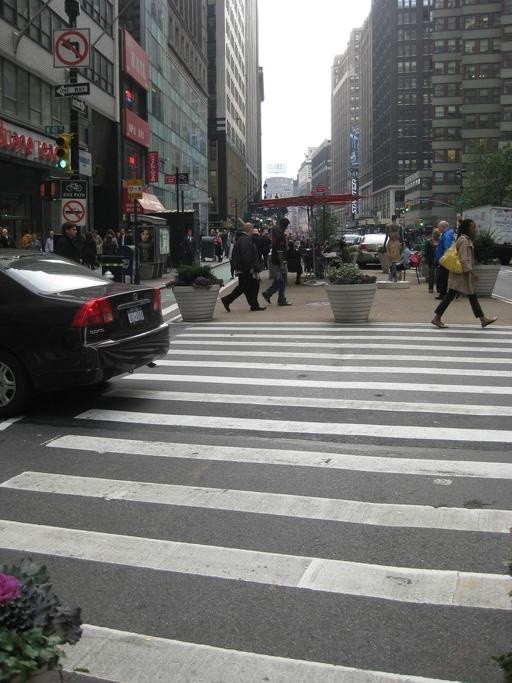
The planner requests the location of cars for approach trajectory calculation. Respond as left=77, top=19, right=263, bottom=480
left=289, top=232, right=389, bottom=269
left=0, top=247, right=169, bottom=414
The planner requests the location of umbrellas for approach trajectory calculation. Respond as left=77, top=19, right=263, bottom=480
left=249, top=191, right=369, bottom=251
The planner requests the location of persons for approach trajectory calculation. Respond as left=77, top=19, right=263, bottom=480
left=389, top=241, right=412, bottom=280
left=424, top=228, right=442, bottom=293
left=430, top=218, right=497, bottom=329
left=434, top=219, right=455, bottom=300
left=385, top=242, right=403, bottom=281
left=381, top=215, right=406, bottom=282
left=261, top=218, right=294, bottom=307
left=220, top=221, right=267, bottom=312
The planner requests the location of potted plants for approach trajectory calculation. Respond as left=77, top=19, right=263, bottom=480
left=169, top=262, right=225, bottom=321
left=324, top=260, right=378, bottom=321
left=471, top=225, right=503, bottom=296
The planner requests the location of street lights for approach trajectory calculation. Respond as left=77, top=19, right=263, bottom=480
left=454, top=167, right=467, bottom=220
left=262, top=179, right=268, bottom=198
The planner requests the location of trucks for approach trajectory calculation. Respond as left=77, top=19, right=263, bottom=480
left=455, top=204, right=512, bottom=265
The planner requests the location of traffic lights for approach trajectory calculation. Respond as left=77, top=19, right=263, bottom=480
left=418, top=218, right=423, bottom=226
left=405, top=202, right=409, bottom=211
left=56, top=132, right=72, bottom=171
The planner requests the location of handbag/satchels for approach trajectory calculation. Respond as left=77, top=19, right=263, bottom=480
left=438, top=235, right=466, bottom=274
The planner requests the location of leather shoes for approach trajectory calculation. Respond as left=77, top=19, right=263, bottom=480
left=278, top=300, right=292, bottom=306
left=221, top=296, right=231, bottom=312
left=431, top=318, right=448, bottom=328
left=251, top=305, right=266, bottom=311
left=261, top=291, right=272, bottom=303
left=480, top=315, right=499, bottom=328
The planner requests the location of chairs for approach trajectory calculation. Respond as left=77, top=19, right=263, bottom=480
left=301, top=253, right=314, bottom=277
left=401, top=253, right=420, bottom=283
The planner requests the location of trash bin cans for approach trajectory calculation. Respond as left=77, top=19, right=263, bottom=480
left=201, top=236, right=217, bottom=262
left=118, top=245, right=136, bottom=284
left=98, top=254, right=130, bottom=283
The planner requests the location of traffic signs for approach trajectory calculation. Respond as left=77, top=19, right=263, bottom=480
left=56, top=81, right=90, bottom=97
